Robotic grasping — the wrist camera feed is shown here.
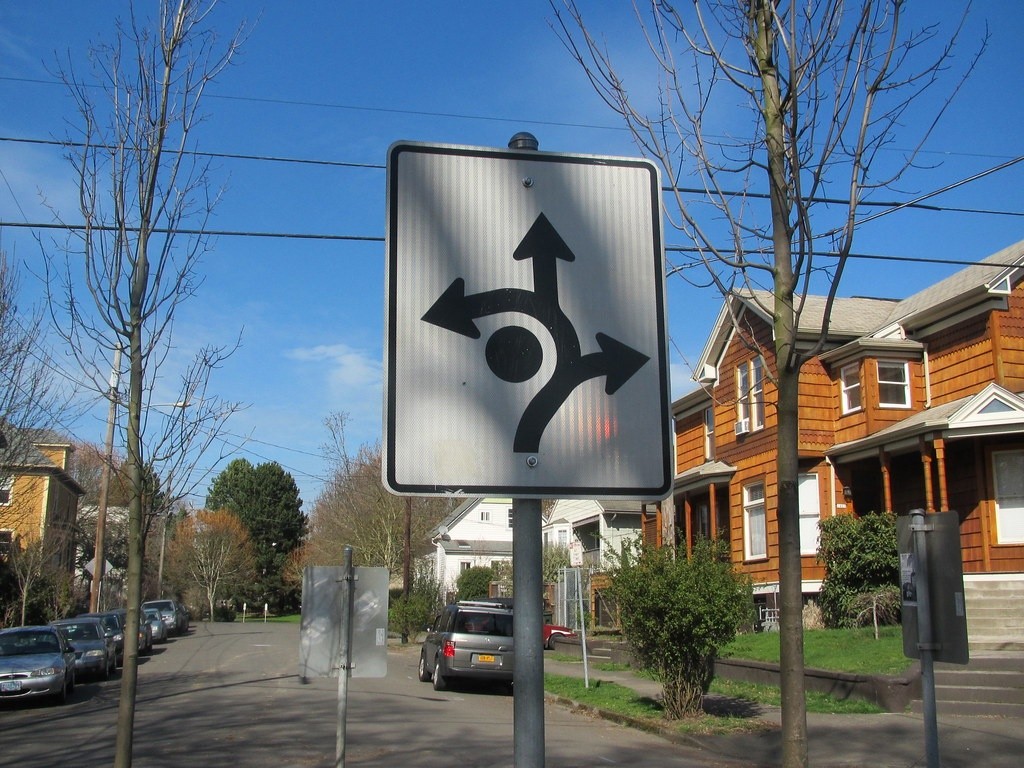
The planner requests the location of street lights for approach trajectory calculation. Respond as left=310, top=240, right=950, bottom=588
left=89, top=402, right=192, bottom=613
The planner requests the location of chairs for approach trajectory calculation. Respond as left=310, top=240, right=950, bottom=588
left=73, top=628, right=85, bottom=638
left=64, top=629, right=69, bottom=636
left=2, top=644, right=18, bottom=653
left=40, top=641, right=55, bottom=649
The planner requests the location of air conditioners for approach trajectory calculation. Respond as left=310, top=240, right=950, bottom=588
left=734, top=419, right=749, bottom=436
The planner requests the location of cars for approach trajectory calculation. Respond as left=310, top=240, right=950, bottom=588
left=42, top=617, right=117, bottom=682
left=1, top=625, right=76, bottom=706
left=110, top=608, right=152, bottom=656
left=418, top=601, right=514, bottom=692
left=543, top=624, right=578, bottom=650
left=144, top=609, right=168, bottom=645
left=141, top=600, right=190, bottom=637
left=76, top=612, right=125, bottom=666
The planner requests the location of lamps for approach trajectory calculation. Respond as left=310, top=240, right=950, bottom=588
left=843, top=485, right=853, bottom=497
